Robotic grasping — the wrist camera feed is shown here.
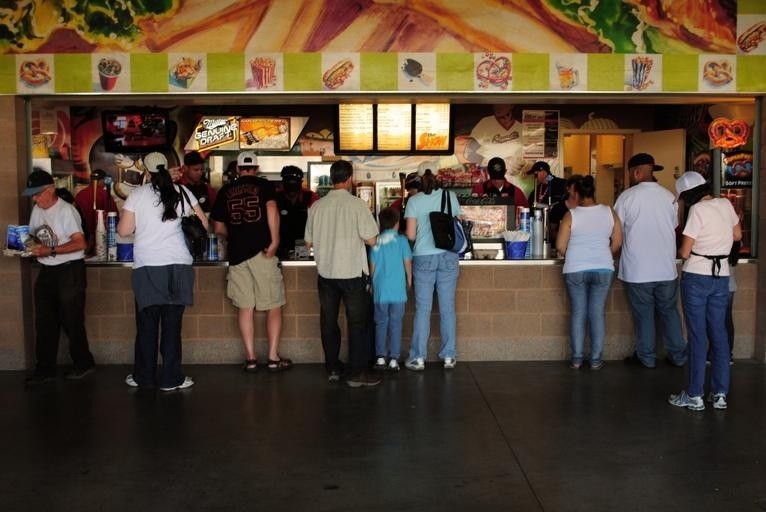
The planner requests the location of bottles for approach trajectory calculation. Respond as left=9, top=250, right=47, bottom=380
left=206, top=233, right=218, bottom=261
left=239, top=123, right=287, bottom=146
left=107, top=211, right=117, bottom=260
left=381, top=186, right=402, bottom=197
left=96, top=210, right=108, bottom=260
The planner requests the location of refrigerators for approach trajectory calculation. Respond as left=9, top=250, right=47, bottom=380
left=32, top=157, right=74, bottom=205
left=683, top=125, right=751, bottom=259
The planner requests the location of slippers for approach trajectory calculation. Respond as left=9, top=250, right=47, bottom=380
left=591, top=361, right=606, bottom=371
left=568, top=362, right=583, bottom=370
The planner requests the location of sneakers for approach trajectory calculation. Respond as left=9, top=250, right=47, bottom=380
left=160, top=376, right=196, bottom=392
left=328, top=357, right=400, bottom=388
left=707, top=391, right=728, bottom=409
left=123, top=374, right=141, bottom=386
left=667, top=389, right=706, bottom=411
left=64, top=358, right=97, bottom=381
left=444, top=356, right=456, bottom=369
left=24, top=371, right=56, bottom=387
left=404, top=357, right=425, bottom=371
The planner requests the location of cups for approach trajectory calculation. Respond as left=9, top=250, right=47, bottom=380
left=6, top=224, right=20, bottom=250
left=516, top=205, right=544, bottom=258
left=720, top=193, right=745, bottom=209
left=97, top=60, right=122, bottom=90
left=14, top=225, right=30, bottom=252
left=556, top=60, right=580, bottom=90
left=319, top=175, right=328, bottom=186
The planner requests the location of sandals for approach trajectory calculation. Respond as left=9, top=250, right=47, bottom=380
left=240, top=359, right=258, bottom=373
left=267, top=359, right=294, bottom=373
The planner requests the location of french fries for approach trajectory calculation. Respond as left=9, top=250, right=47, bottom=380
left=630, top=56, right=652, bottom=89
left=249, top=57, right=275, bottom=87
left=175, top=60, right=197, bottom=83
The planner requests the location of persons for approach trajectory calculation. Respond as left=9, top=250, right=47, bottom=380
left=117, top=151, right=318, bottom=390
left=304, top=158, right=463, bottom=391
left=75, top=170, right=121, bottom=258
left=22, top=169, right=97, bottom=386
left=471, top=157, right=573, bottom=227
left=553, top=152, right=742, bottom=410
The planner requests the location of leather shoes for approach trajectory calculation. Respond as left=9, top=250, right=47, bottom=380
left=624, top=356, right=648, bottom=369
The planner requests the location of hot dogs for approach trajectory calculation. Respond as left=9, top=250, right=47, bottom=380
left=738, top=20, right=766, bottom=52
left=323, top=58, right=353, bottom=89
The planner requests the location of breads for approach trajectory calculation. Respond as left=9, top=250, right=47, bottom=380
left=709, top=117, right=750, bottom=150
left=21, top=61, right=51, bottom=85
left=705, top=60, right=733, bottom=86
left=724, top=153, right=752, bottom=165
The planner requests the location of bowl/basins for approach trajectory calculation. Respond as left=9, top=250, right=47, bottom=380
left=472, top=249, right=498, bottom=260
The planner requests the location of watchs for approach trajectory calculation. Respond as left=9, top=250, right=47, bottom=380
left=50, top=247, right=56, bottom=257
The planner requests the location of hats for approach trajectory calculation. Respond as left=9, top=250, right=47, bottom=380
left=20, top=170, right=54, bottom=198
left=488, top=157, right=506, bottom=180
left=142, top=151, right=168, bottom=173
left=526, top=161, right=550, bottom=175
left=672, top=171, right=707, bottom=206
left=236, top=150, right=258, bottom=167
left=405, top=173, right=420, bottom=189
left=91, top=169, right=112, bottom=180
left=417, top=161, right=440, bottom=177
left=627, top=153, right=665, bottom=172
left=184, top=151, right=206, bottom=165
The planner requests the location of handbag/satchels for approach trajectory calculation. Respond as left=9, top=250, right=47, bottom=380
left=429, top=188, right=473, bottom=255
left=175, top=182, right=208, bottom=258
